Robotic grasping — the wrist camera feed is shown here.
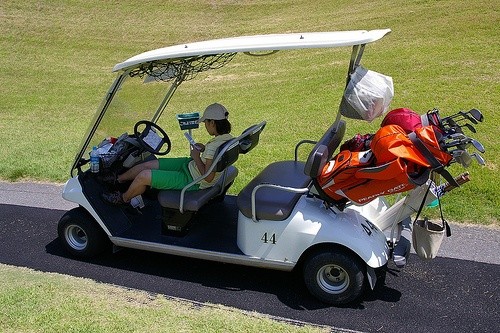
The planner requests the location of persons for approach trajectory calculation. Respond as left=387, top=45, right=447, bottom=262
left=99, top=103, right=234, bottom=208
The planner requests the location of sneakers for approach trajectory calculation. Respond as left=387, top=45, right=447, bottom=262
left=102, top=190, right=131, bottom=207
left=97, top=173, right=117, bottom=184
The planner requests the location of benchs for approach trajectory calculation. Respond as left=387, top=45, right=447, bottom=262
left=236, top=119, right=346, bottom=221
left=159, top=120, right=267, bottom=222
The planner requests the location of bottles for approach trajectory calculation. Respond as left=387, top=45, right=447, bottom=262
left=90, top=146, right=99, bottom=172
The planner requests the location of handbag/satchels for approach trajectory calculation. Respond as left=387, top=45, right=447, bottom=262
left=413, top=180, right=451, bottom=262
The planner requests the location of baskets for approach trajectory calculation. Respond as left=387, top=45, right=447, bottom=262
left=89, top=139, right=128, bottom=168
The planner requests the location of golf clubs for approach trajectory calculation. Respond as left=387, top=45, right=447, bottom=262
left=442, top=109, right=486, bottom=168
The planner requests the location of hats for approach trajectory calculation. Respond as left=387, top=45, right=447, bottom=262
left=196, top=103, right=228, bottom=123
